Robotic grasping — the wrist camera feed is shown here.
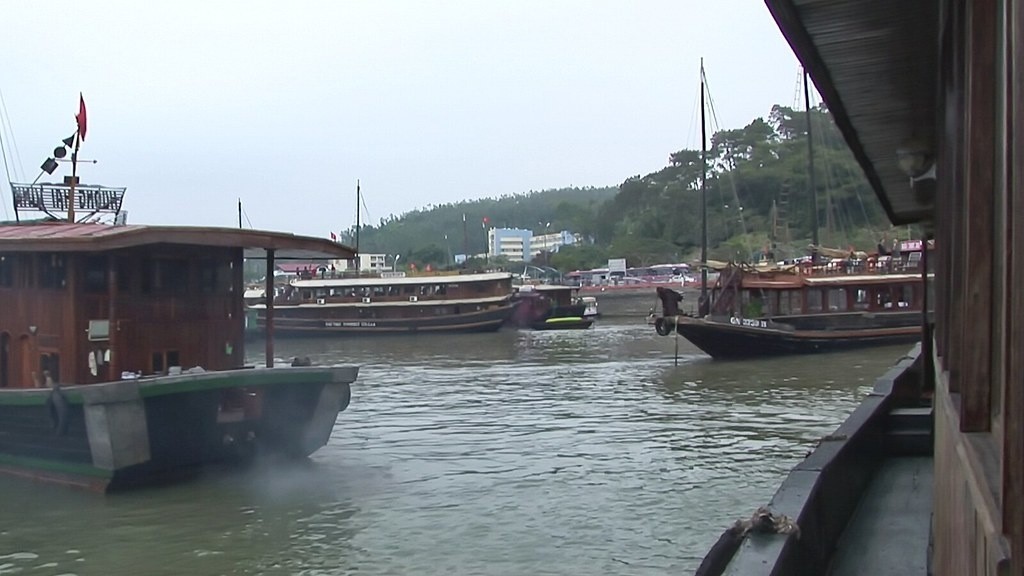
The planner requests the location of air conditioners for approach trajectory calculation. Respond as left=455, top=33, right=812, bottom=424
left=361, top=296, right=371, bottom=304
left=316, top=298, right=326, bottom=305
left=409, top=295, right=418, bottom=302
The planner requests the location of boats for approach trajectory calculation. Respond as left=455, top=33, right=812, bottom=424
left=510, top=282, right=595, bottom=331
left=0, top=170, right=361, bottom=499
left=643, top=54, right=937, bottom=359
left=241, top=255, right=524, bottom=338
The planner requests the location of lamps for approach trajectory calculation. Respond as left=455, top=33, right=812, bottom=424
left=894, top=117, right=936, bottom=191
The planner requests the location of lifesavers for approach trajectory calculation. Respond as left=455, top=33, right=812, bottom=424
left=800, top=259, right=813, bottom=277
left=655, top=318, right=672, bottom=336
left=866, top=257, right=875, bottom=270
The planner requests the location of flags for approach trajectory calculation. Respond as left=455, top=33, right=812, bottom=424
left=76, top=97, right=88, bottom=141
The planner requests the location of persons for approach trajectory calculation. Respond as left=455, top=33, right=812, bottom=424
left=891, top=238, right=902, bottom=257
left=878, top=238, right=889, bottom=256
left=847, top=251, right=858, bottom=260
